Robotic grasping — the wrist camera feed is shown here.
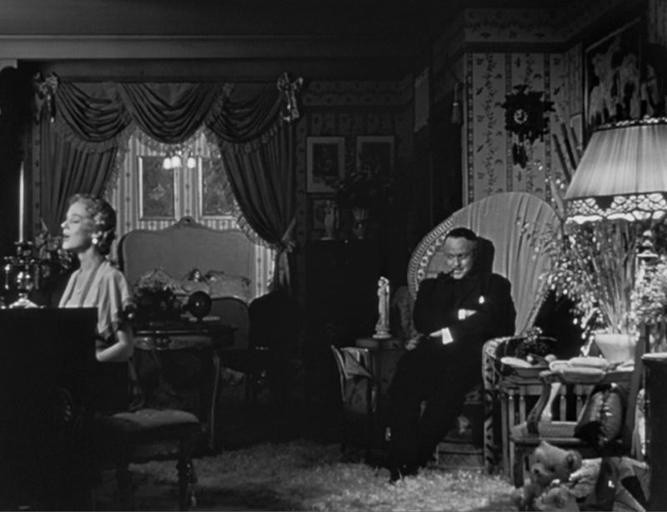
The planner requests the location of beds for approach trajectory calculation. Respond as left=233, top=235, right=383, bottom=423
left=116, top=218, right=262, bottom=309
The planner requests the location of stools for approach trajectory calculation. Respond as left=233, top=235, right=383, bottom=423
left=42, top=383, right=201, bottom=511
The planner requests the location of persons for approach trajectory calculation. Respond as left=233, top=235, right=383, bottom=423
left=378, top=226, right=501, bottom=483
left=0, top=192, right=139, bottom=417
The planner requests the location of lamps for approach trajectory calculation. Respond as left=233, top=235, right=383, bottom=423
left=561, top=116, right=664, bottom=466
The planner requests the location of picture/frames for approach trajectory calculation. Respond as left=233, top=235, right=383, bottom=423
left=353, top=133, right=397, bottom=188
left=304, top=131, right=349, bottom=245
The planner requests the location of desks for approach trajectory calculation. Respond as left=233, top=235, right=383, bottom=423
left=128, top=322, right=234, bottom=452
left=498, top=375, right=567, bottom=485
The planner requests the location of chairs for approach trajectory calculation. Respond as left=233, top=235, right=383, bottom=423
left=395, top=189, right=568, bottom=479
left=509, top=331, right=648, bottom=511
